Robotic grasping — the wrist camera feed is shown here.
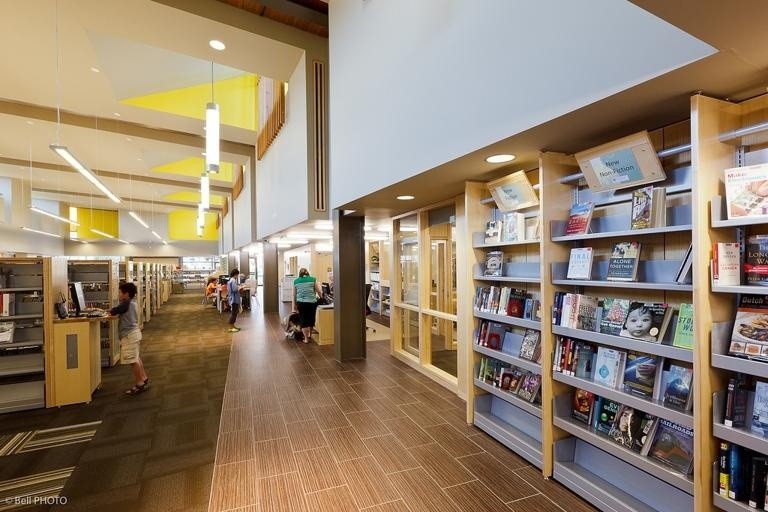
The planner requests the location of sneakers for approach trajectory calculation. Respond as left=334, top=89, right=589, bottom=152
left=145, top=377, right=152, bottom=385
left=228, top=327, right=240, bottom=332
left=125, top=383, right=149, bottom=395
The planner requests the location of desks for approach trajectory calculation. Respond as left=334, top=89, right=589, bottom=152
left=215, top=286, right=250, bottom=313
left=311, top=306, right=336, bottom=345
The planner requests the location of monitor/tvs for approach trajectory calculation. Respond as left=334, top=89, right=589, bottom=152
left=71, top=281, right=87, bottom=317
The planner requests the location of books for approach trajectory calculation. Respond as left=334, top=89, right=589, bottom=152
left=568, top=247, right=594, bottom=280
left=564, top=201, right=596, bottom=235
left=0, top=294, right=17, bottom=316
left=713, top=163, right=768, bottom=511
left=552, top=291, right=694, bottom=480
left=607, top=241, right=642, bottom=281
left=0, top=322, right=15, bottom=343
left=631, top=186, right=666, bottom=230
left=474, top=213, right=542, bottom=405
left=675, top=242, right=692, bottom=285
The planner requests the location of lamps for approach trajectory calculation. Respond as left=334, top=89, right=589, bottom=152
left=201, top=169, right=209, bottom=211
left=27, top=142, right=80, bottom=227
left=88, top=186, right=115, bottom=239
left=149, top=195, right=169, bottom=247
left=20, top=169, right=62, bottom=239
left=127, top=175, right=151, bottom=231
left=117, top=197, right=131, bottom=245
left=49, top=1, right=123, bottom=204
left=204, top=61, right=220, bottom=174
left=197, top=216, right=202, bottom=238
left=199, top=202, right=205, bottom=229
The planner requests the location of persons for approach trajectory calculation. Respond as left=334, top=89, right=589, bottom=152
left=228, top=269, right=246, bottom=332
left=206, top=277, right=217, bottom=306
left=110, top=281, right=149, bottom=394
left=220, top=278, right=229, bottom=311
left=292, top=267, right=322, bottom=343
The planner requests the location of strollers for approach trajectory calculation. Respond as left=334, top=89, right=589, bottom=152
left=281, top=311, right=307, bottom=341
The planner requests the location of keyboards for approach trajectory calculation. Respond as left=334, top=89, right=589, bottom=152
left=88, top=310, right=108, bottom=316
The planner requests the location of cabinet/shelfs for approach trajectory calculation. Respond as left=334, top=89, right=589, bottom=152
left=465, top=89, right=768, bottom=512
left=0, top=252, right=68, bottom=414
left=174, top=269, right=214, bottom=290
left=371, top=240, right=391, bottom=318
left=118, top=261, right=172, bottom=330
left=67, top=259, right=120, bottom=367
left=53, top=312, right=120, bottom=406
